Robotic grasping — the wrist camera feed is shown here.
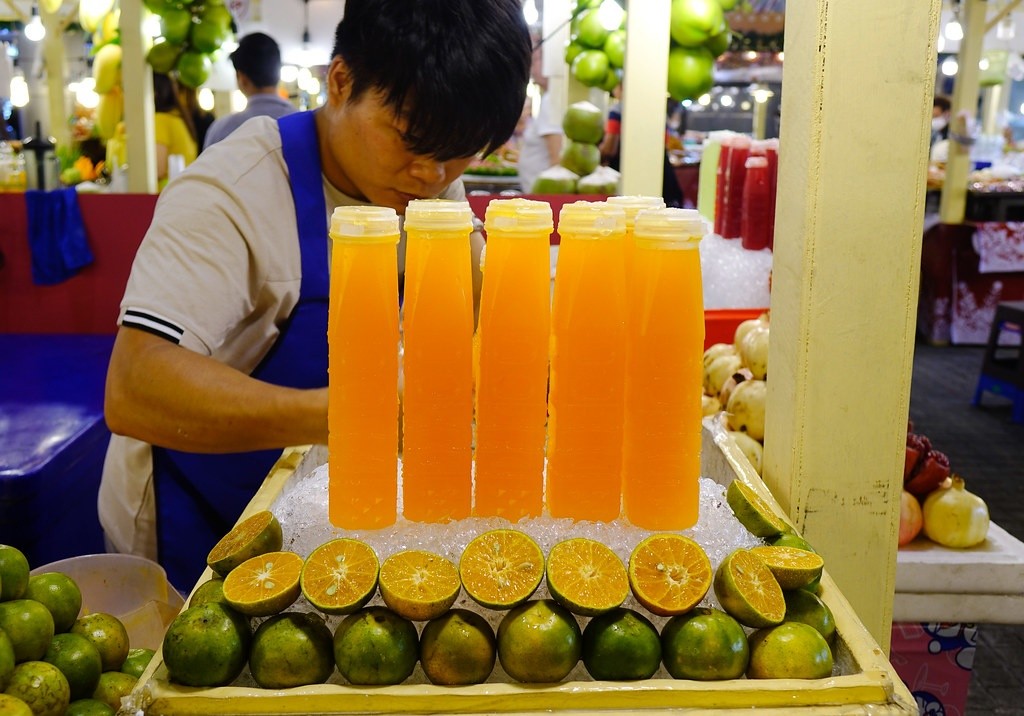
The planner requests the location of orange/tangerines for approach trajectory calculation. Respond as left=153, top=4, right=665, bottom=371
left=162, top=479, right=836, bottom=687
left=0, top=545, right=160, bottom=716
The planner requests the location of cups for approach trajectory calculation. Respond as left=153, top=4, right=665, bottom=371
left=167, top=154, right=184, bottom=180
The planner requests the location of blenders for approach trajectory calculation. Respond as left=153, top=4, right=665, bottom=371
left=22, top=137, right=58, bottom=192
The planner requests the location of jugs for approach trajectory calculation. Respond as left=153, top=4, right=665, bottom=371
left=30, top=554, right=187, bottom=652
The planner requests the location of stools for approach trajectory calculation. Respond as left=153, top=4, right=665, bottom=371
left=970, top=302, right=1024, bottom=425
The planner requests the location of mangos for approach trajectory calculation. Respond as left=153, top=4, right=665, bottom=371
left=73, top=1, right=128, bottom=173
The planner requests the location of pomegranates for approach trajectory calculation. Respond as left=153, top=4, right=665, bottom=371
left=701, top=309, right=989, bottom=550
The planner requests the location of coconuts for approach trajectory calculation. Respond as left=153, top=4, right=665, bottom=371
left=533, top=100, right=622, bottom=194
left=138, top=1, right=234, bottom=86
left=567, top=1, right=743, bottom=104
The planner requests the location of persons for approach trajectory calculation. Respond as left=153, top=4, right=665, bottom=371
left=74, top=32, right=303, bottom=207
left=97, top=0, right=533, bottom=594
left=510, top=21, right=684, bottom=208
left=929, top=97, right=952, bottom=156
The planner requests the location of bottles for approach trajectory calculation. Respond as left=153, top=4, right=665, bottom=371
left=402, top=199, right=475, bottom=525
left=697, top=137, right=720, bottom=222
left=748, top=141, right=767, bottom=157
left=714, top=141, right=730, bottom=235
left=326, top=205, right=399, bottom=532
left=547, top=201, right=628, bottom=524
left=607, top=194, right=666, bottom=494
left=474, top=199, right=554, bottom=519
left=742, top=157, right=769, bottom=251
left=623, top=208, right=705, bottom=532
left=766, top=137, right=779, bottom=251
left=722, top=140, right=750, bottom=239
